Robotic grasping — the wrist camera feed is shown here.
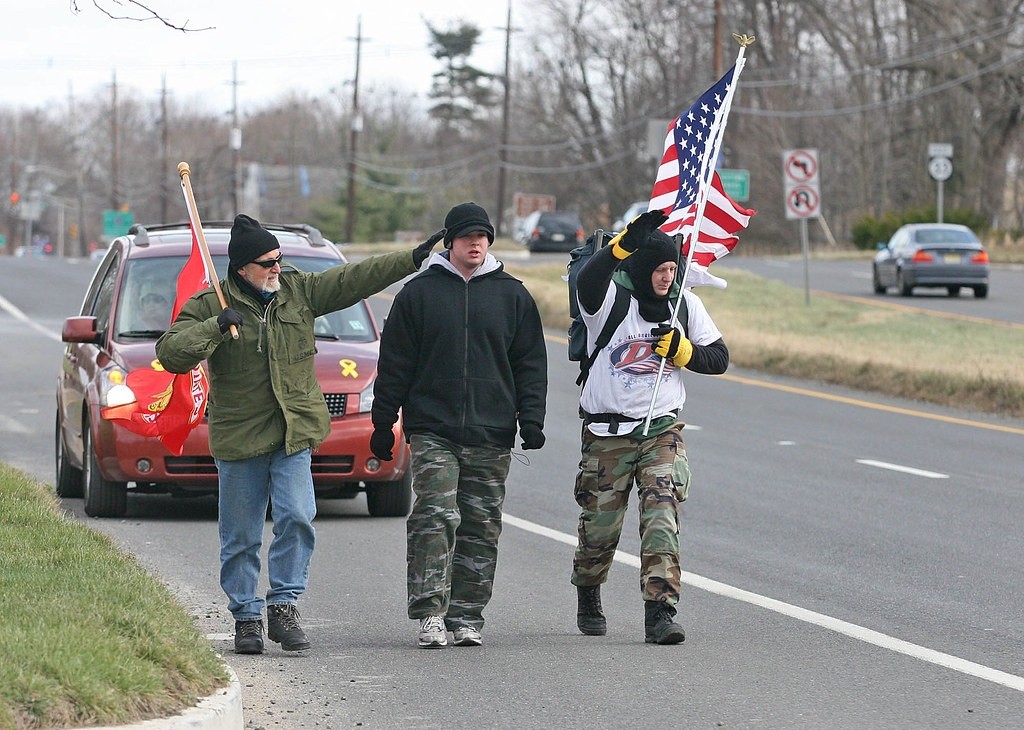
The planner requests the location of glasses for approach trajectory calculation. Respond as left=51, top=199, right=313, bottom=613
left=251, top=251, right=283, bottom=268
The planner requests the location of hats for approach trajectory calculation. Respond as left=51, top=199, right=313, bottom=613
left=444, top=203, right=494, bottom=249
left=228, top=214, right=280, bottom=271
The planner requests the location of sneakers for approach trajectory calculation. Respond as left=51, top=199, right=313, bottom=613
left=645, top=601, right=685, bottom=644
left=577, top=585, right=607, bottom=635
left=234, top=618, right=265, bottom=654
left=420, top=616, right=448, bottom=648
left=454, top=627, right=481, bottom=646
left=267, top=606, right=310, bottom=650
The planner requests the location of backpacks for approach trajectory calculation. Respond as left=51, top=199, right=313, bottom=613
left=566, top=229, right=689, bottom=362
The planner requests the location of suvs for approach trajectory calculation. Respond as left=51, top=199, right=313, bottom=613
left=53, top=218, right=414, bottom=519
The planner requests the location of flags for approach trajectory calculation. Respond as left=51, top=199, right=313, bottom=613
left=647, top=61, right=757, bottom=293
left=100, top=219, right=210, bottom=459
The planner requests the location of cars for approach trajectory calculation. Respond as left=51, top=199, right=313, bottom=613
left=611, top=202, right=651, bottom=233
left=872, top=222, right=989, bottom=299
left=522, top=208, right=585, bottom=256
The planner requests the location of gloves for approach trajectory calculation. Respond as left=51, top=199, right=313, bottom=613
left=609, top=210, right=669, bottom=259
left=217, top=307, right=243, bottom=335
left=520, top=422, right=545, bottom=450
left=651, top=324, right=693, bottom=367
left=369, top=430, right=395, bottom=462
left=413, top=229, right=446, bottom=265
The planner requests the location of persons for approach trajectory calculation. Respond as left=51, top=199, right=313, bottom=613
left=570, top=210, right=729, bottom=645
left=368, top=202, right=548, bottom=645
left=153, top=207, right=446, bottom=656
